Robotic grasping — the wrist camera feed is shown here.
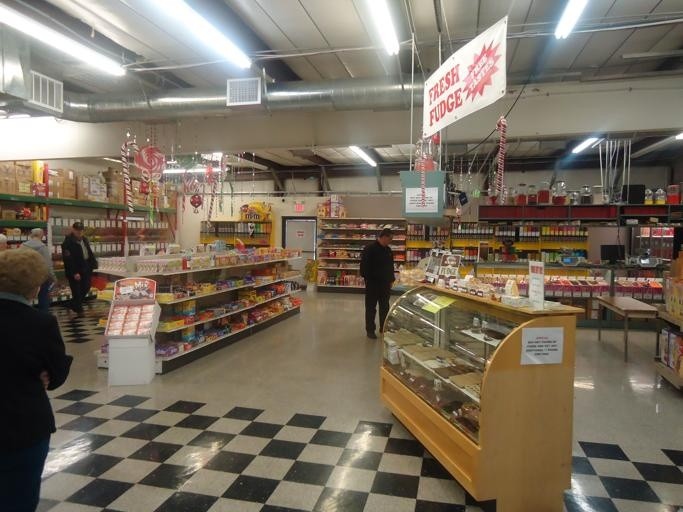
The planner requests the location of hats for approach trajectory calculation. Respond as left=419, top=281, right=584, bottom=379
left=73, top=222, right=84, bottom=229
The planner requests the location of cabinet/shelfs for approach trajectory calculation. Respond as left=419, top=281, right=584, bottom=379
left=653, top=310, right=683, bottom=391
left=378, top=284, right=586, bottom=512
left=0, top=193, right=176, bottom=278
left=476, top=202, right=683, bottom=227
left=475, top=259, right=675, bottom=332
left=93, top=257, right=302, bottom=375
left=199, top=220, right=271, bottom=248
left=316, top=219, right=406, bottom=292
left=406, top=223, right=590, bottom=277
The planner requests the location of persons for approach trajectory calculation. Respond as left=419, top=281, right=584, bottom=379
left=0, top=248, right=73, bottom=512
left=0, top=233, right=8, bottom=251
left=61, top=222, right=98, bottom=312
left=19, top=228, right=57, bottom=313
left=361, top=228, right=395, bottom=338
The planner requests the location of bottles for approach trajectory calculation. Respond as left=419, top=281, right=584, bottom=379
left=407, top=248, right=422, bottom=262
left=218, top=222, right=269, bottom=233
left=645, top=184, right=680, bottom=204
left=325, top=275, right=343, bottom=286
left=451, top=221, right=589, bottom=263
left=487, top=179, right=604, bottom=206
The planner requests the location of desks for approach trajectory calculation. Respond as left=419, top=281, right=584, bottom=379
left=598, top=296, right=659, bottom=362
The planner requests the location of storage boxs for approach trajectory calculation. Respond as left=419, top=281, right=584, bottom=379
left=0, top=161, right=175, bottom=209
